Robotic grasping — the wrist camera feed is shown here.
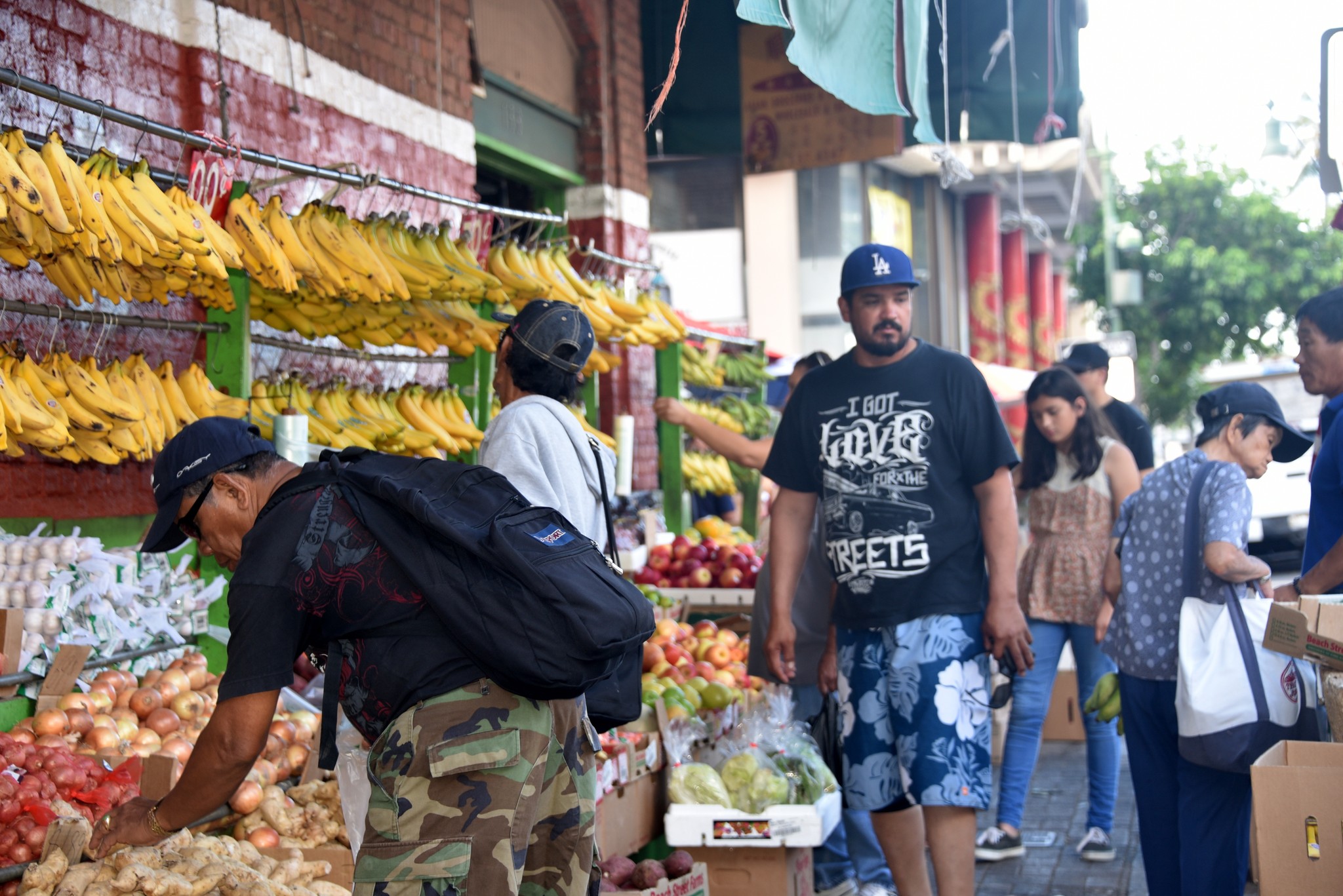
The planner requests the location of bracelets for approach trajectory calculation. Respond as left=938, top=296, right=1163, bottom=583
left=1257, top=570, right=1274, bottom=584
left=1291, top=575, right=1306, bottom=597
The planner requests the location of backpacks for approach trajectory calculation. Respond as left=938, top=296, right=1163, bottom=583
left=254, top=447, right=657, bottom=772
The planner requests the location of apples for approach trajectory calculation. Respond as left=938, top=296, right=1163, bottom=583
left=604, top=526, right=778, bottom=724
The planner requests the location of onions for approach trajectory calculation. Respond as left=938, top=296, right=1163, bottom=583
left=1, top=645, right=330, bottom=896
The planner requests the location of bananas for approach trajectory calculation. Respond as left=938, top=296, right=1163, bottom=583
left=0, top=120, right=786, bottom=503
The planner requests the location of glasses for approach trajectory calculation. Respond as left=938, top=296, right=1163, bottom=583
left=177, top=465, right=246, bottom=542
left=1073, top=366, right=1104, bottom=374
left=497, top=328, right=512, bottom=340
left=965, top=648, right=1016, bottom=708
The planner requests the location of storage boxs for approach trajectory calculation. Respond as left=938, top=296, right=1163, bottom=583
left=687, top=842, right=815, bottom=896
left=1244, top=600, right=1343, bottom=896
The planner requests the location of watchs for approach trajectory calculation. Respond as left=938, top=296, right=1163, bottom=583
left=146, top=793, right=180, bottom=838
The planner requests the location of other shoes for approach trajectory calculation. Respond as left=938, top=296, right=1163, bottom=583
left=812, top=879, right=893, bottom=896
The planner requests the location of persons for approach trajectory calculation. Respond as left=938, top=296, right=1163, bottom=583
left=960, top=361, right=1144, bottom=865
left=1099, top=376, right=1322, bottom=896
left=54, top=416, right=647, bottom=896
left=648, top=347, right=844, bottom=731
left=1266, top=289, right=1343, bottom=599
left=482, top=294, right=625, bottom=562
left=1055, top=339, right=1161, bottom=477
left=768, top=240, right=1036, bottom=896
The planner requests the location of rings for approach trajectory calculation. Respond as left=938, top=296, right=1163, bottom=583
left=101, top=813, right=112, bottom=832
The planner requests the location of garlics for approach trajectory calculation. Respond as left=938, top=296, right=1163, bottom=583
left=0, top=516, right=212, bottom=679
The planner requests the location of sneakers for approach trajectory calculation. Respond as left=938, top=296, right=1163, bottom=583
left=973, top=826, right=1024, bottom=860
left=1075, top=826, right=1115, bottom=860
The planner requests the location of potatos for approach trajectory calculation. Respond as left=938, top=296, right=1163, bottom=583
left=596, top=830, right=695, bottom=891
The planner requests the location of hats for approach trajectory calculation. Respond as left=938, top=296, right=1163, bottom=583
left=1195, top=381, right=1314, bottom=463
left=491, top=298, right=595, bottom=374
left=138, top=415, right=276, bottom=554
left=840, top=243, right=920, bottom=292
left=1052, top=343, right=1110, bottom=371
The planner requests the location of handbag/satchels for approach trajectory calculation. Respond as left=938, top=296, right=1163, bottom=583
left=1178, top=460, right=1321, bottom=770
left=587, top=436, right=644, bottom=734
